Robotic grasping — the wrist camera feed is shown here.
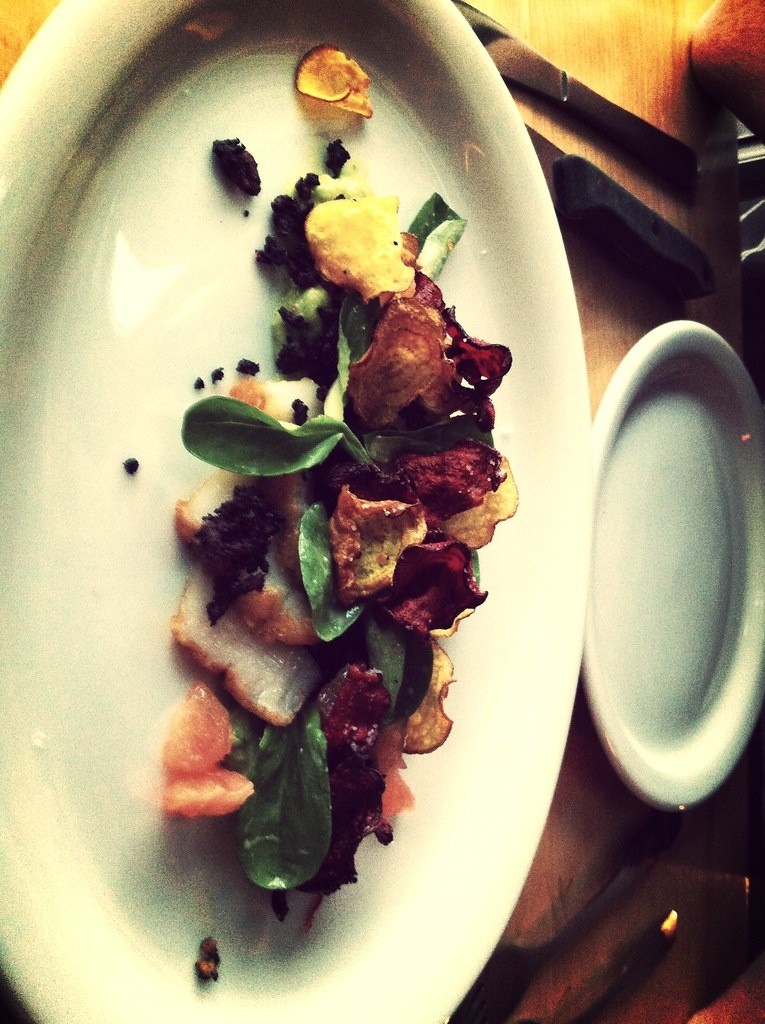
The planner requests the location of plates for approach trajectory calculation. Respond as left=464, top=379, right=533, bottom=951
left=5, top=5, right=579, bottom=1024
left=582, top=320, right=764, bottom=808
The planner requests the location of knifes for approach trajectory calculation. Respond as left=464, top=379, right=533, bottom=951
left=454, top=1, right=713, bottom=191
left=524, top=124, right=718, bottom=301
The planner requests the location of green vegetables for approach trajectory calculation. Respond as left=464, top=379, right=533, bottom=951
left=182, top=193, right=469, bottom=889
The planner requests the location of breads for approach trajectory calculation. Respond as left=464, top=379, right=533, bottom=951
left=691, top=0, right=765, bottom=148
